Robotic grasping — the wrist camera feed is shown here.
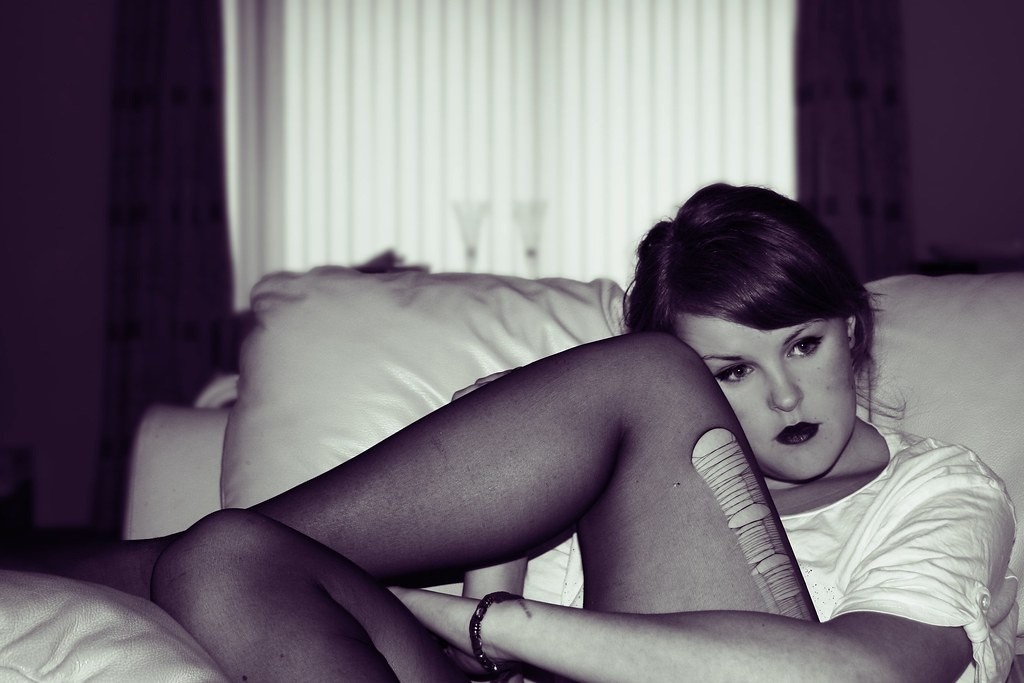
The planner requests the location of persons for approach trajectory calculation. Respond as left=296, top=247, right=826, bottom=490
left=49, top=182, right=1024, bottom=683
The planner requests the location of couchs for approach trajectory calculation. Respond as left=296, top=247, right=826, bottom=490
left=0, top=264, right=1024, bottom=682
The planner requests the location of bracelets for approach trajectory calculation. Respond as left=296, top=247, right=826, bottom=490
left=469, top=590, right=526, bottom=679
